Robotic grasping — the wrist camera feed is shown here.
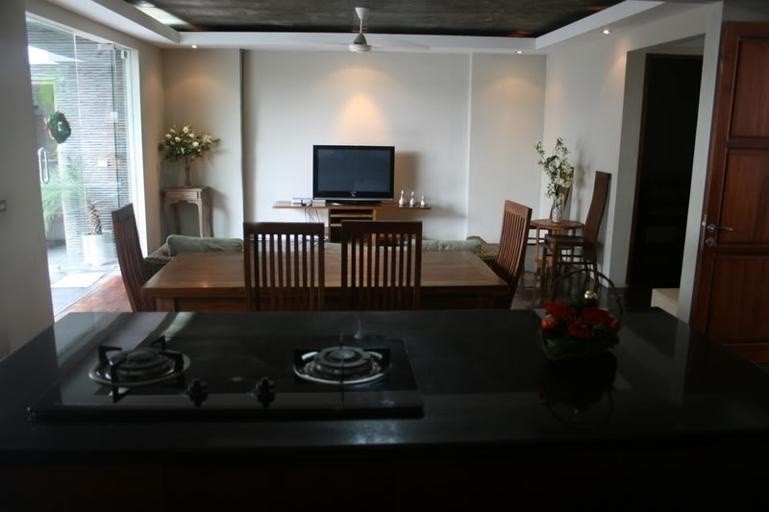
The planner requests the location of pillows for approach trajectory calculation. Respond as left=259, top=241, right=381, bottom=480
left=166, top=235, right=243, bottom=257
left=409, top=238, right=482, bottom=255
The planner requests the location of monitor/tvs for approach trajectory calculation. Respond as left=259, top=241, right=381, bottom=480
left=313, top=145, right=394, bottom=206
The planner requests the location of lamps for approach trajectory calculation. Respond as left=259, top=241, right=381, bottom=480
left=348, top=7, right=370, bottom=52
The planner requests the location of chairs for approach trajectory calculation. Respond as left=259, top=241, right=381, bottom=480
left=110, top=202, right=151, bottom=312
left=541, top=170, right=611, bottom=298
left=340, top=219, right=422, bottom=309
left=243, top=220, right=325, bottom=311
left=490, top=199, right=533, bottom=308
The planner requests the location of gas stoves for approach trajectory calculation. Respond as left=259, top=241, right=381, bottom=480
left=25, top=330, right=427, bottom=424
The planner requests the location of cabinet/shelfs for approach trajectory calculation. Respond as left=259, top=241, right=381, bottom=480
left=271, top=200, right=432, bottom=244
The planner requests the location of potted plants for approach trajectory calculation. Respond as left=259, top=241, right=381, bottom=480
left=37, top=156, right=113, bottom=266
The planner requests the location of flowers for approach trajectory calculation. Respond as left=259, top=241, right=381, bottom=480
left=533, top=136, right=576, bottom=208
left=156, top=126, right=222, bottom=167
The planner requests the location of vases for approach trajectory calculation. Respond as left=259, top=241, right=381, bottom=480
left=182, top=153, right=195, bottom=187
left=551, top=196, right=563, bottom=223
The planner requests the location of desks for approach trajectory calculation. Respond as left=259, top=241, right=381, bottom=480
left=140, top=250, right=508, bottom=311
left=530, top=218, right=585, bottom=303
left=161, top=187, right=212, bottom=240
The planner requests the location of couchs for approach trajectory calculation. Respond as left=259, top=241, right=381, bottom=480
left=144, top=233, right=498, bottom=263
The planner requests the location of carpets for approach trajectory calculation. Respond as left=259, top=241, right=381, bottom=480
left=51, top=270, right=108, bottom=290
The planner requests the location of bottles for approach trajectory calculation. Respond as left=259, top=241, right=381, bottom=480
left=410, top=192, right=415, bottom=206
left=398, top=189, right=407, bottom=206
left=420, top=195, right=426, bottom=207
